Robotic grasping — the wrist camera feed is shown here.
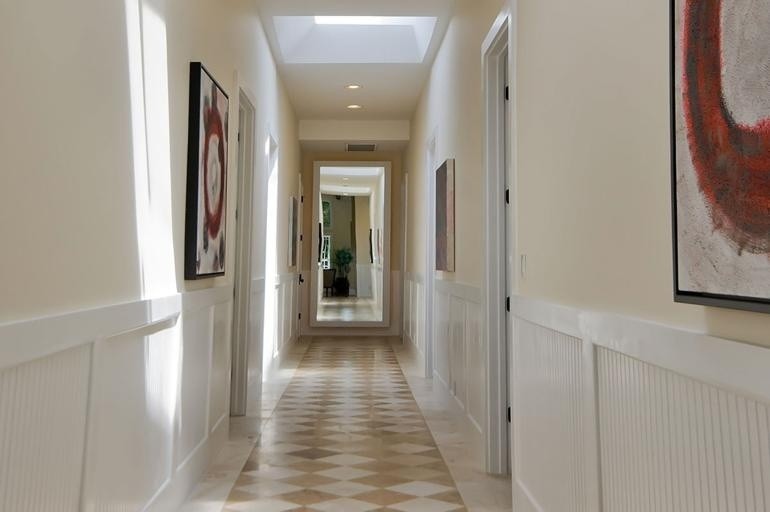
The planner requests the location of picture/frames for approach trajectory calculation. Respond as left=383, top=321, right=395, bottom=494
left=183, top=60, right=230, bottom=283
left=288, top=195, right=298, bottom=269
left=434, top=157, right=456, bottom=273
left=669, top=0, right=770, bottom=304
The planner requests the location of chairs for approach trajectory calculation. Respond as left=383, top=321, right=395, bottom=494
left=323, top=269, right=336, bottom=298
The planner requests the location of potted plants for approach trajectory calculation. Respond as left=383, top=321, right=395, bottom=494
left=334, top=248, right=355, bottom=296
